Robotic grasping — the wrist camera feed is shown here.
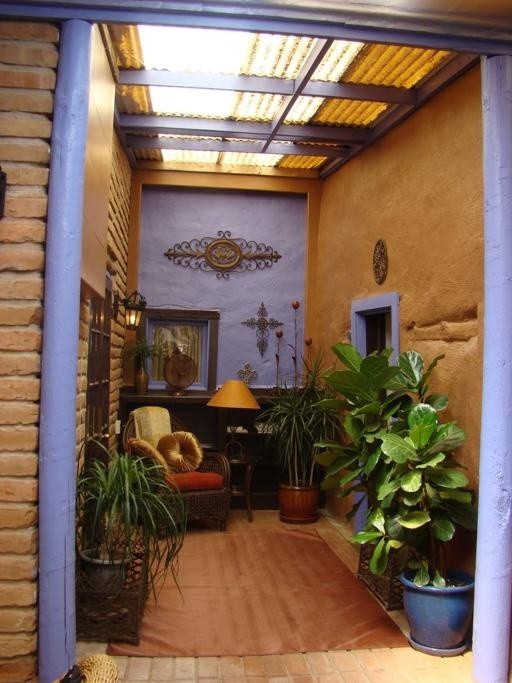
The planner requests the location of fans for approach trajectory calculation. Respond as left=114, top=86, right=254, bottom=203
left=163, top=353, right=197, bottom=396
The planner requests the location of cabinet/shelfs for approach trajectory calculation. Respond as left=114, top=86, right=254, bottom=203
left=121, top=390, right=325, bottom=510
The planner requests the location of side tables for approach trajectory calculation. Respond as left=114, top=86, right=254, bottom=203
left=219, top=454, right=264, bottom=522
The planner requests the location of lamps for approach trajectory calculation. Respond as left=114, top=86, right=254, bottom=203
left=114, top=290, right=147, bottom=329
left=205, top=380, right=261, bottom=461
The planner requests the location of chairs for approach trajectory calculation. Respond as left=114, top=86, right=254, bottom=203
left=122, top=405, right=231, bottom=541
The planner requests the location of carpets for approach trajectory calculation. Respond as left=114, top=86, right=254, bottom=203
left=105, top=511, right=411, bottom=657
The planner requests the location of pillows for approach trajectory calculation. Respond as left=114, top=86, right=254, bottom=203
left=157, top=430, right=205, bottom=473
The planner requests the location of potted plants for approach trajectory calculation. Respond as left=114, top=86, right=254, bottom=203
left=348, top=402, right=474, bottom=655
left=310, top=342, right=449, bottom=611
left=124, top=341, right=160, bottom=396
left=254, top=347, right=344, bottom=524
left=77, top=434, right=189, bottom=648
